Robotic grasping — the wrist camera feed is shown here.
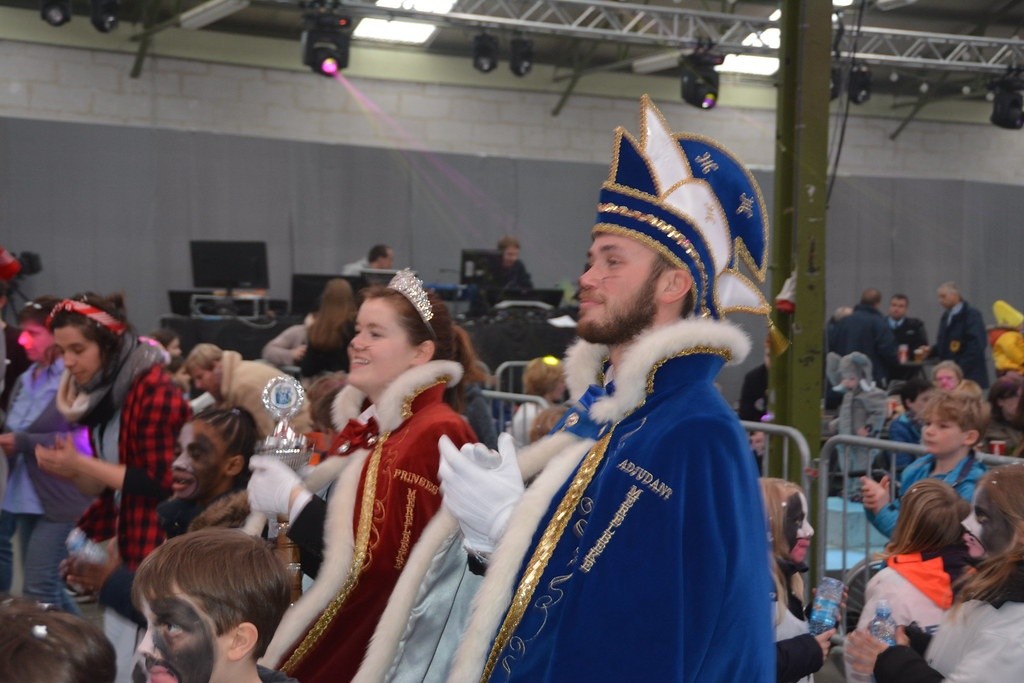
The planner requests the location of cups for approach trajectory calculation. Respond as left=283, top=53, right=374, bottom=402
left=914, top=349, right=923, bottom=362
left=887, top=398, right=896, bottom=416
left=898, top=344, right=908, bottom=363
left=989, top=441, right=1006, bottom=456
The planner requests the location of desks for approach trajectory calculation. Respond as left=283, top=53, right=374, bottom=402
left=161, top=313, right=577, bottom=395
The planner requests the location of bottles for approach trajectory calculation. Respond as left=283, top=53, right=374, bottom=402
left=65, top=527, right=106, bottom=565
left=808, top=576, right=845, bottom=635
left=867, top=598, right=896, bottom=647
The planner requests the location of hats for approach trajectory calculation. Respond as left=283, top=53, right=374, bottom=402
left=589, top=90, right=790, bottom=355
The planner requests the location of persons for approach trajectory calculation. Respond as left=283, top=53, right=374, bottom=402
left=185, top=344, right=314, bottom=452
left=130, top=527, right=300, bottom=683
left=861, top=378, right=995, bottom=547
left=262, top=244, right=395, bottom=370
left=155, top=404, right=276, bottom=549
left=843, top=463, right=1024, bottom=683
left=845, top=479, right=985, bottom=683
left=739, top=282, right=1024, bottom=478
left=303, top=369, right=356, bottom=504
left=350, top=95, right=772, bottom=683
left=246, top=284, right=481, bottom=683
left=509, top=353, right=566, bottom=448
left=0, top=274, right=192, bottom=622
left=471, top=234, right=533, bottom=308
left=755, top=477, right=848, bottom=683
left=0, top=598, right=118, bottom=683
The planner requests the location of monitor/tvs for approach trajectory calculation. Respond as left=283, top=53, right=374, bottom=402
left=497, top=290, right=566, bottom=308
left=290, top=272, right=361, bottom=316
left=191, top=240, right=269, bottom=297
left=461, top=249, right=497, bottom=284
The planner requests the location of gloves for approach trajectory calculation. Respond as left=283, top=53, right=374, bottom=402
left=439, top=431, right=526, bottom=554
left=248, top=455, right=303, bottom=514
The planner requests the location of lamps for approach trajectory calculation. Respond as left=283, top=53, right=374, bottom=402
left=89, top=0, right=118, bottom=32
left=681, top=37, right=724, bottom=109
left=296, top=0, right=351, bottom=77
left=40, top=0, right=73, bottom=26
left=508, top=31, right=533, bottom=75
left=830, top=56, right=873, bottom=105
left=472, top=26, right=499, bottom=70
left=986, top=64, right=1024, bottom=129
